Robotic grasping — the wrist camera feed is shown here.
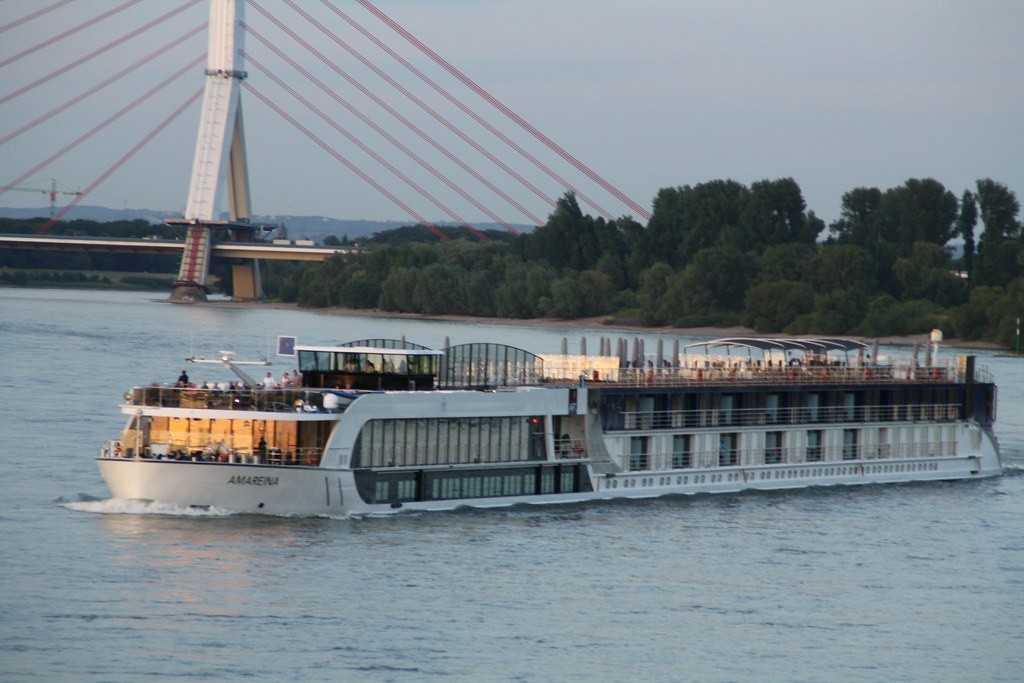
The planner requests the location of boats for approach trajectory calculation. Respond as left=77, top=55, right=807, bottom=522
left=98, top=310, right=1004, bottom=520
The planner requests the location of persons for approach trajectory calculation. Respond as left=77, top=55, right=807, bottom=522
left=138, top=436, right=279, bottom=464
left=175, top=371, right=303, bottom=410
left=343, top=358, right=391, bottom=378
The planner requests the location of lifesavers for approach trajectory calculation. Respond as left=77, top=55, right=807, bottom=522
left=307, top=449, right=317, bottom=464
